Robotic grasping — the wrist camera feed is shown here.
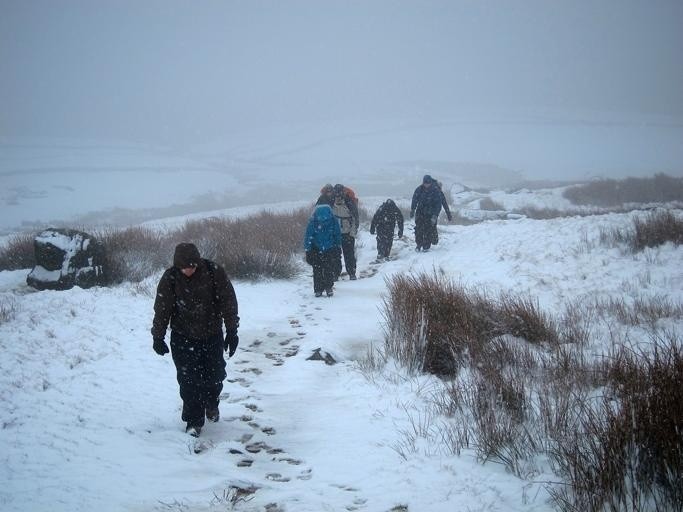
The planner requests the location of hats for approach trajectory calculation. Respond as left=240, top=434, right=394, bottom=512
left=333, top=184, right=343, bottom=194
left=174, top=243, right=200, bottom=270
left=324, top=183, right=333, bottom=192
left=385, top=199, right=394, bottom=208
left=423, top=174, right=433, bottom=184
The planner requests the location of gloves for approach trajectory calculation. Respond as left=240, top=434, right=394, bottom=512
left=397, top=230, right=403, bottom=238
left=305, top=251, right=314, bottom=267
left=370, top=225, right=375, bottom=234
left=448, top=215, right=452, bottom=221
left=224, top=332, right=239, bottom=357
left=152, top=338, right=170, bottom=355
left=409, top=209, right=415, bottom=218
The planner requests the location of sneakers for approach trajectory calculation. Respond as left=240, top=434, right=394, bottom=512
left=314, top=272, right=356, bottom=297
left=415, top=239, right=436, bottom=252
left=206, top=406, right=220, bottom=423
left=377, top=253, right=389, bottom=259
left=185, top=424, right=202, bottom=437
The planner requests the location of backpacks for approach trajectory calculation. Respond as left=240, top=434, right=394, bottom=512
left=311, top=225, right=334, bottom=251
left=343, top=187, right=358, bottom=209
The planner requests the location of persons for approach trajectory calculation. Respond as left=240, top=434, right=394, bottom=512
left=304, top=195, right=341, bottom=297
left=409, top=175, right=441, bottom=253
left=368, top=199, right=403, bottom=259
left=429, top=183, right=450, bottom=245
left=312, top=183, right=334, bottom=207
left=150, top=241, right=238, bottom=435
left=330, top=184, right=358, bottom=280
